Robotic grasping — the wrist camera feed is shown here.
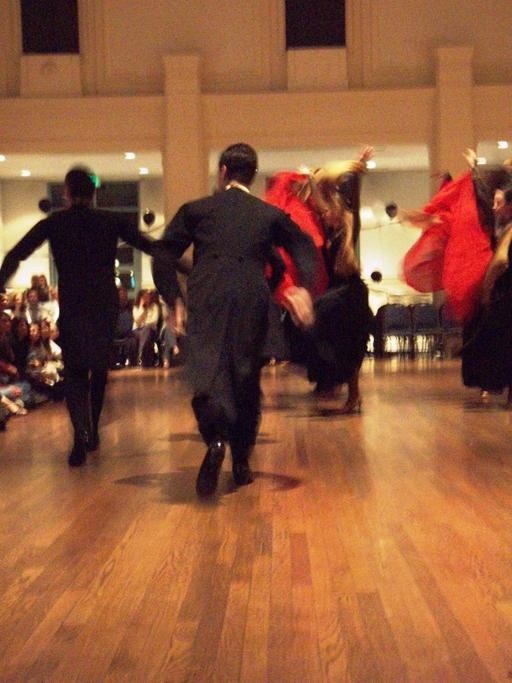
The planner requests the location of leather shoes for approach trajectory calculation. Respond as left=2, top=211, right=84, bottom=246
left=233, top=453, right=251, bottom=484
left=69, top=429, right=98, bottom=465
left=196, top=439, right=225, bottom=496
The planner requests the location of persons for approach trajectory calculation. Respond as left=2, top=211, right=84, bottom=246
left=1, top=163, right=192, bottom=465
left=152, top=140, right=320, bottom=496
left=392, top=147, right=511, bottom=411
left=260, top=144, right=377, bottom=419
left=1, top=270, right=188, bottom=435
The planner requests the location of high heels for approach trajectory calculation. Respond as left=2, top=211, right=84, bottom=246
left=338, top=396, right=361, bottom=414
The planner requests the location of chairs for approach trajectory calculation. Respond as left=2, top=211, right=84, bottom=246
left=375, top=303, right=471, bottom=356
